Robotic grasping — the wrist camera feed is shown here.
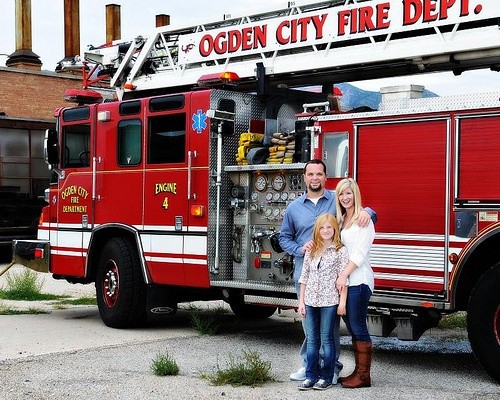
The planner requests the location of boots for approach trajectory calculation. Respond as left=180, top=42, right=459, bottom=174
left=338, top=339, right=372, bottom=388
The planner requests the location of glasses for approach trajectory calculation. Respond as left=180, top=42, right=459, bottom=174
left=317, top=257, right=324, bottom=270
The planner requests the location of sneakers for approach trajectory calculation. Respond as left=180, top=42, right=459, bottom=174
left=332, top=372, right=338, bottom=384
left=290, top=367, right=306, bottom=380
left=298, top=378, right=318, bottom=389
left=313, top=379, right=332, bottom=389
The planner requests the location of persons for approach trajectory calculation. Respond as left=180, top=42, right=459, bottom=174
left=279, top=159, right=378, bottom=386
left=297, top=213, right=349, bottom=390
left=335, top=178, right=375, bottom=389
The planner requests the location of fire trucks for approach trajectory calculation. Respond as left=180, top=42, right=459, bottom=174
left=12, top=0, right=499, bottom=382
